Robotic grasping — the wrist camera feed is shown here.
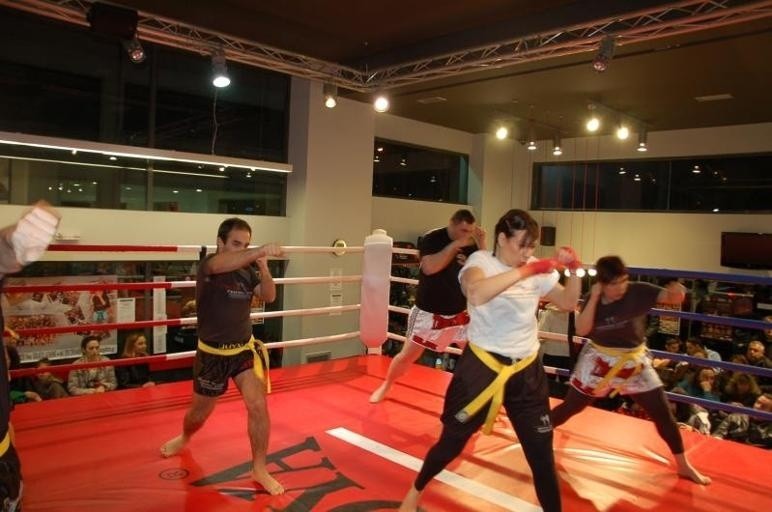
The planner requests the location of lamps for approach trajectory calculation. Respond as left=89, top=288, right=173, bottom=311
left=617, top=163, right=727, bottom=185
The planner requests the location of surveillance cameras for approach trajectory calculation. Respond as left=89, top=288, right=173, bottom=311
left=520, top=139, right=527, bottom=145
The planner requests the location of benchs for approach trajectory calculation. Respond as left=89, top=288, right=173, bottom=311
left=56, top=357, right=194, bottom=386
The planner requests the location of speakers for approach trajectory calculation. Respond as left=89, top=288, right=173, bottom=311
left=87, top=2, right=138, bottom=40
left=540, top=227, right=555, bottom=246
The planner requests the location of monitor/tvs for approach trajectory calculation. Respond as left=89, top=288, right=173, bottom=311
left=720, top=232, right=772, bottom=270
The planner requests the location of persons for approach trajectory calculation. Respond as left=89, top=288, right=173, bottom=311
left=619, top=278, right=772, bottom=449
left=552, top=256, right=713, bottom=485
left=161, top=217, right=286, bottom=497
left=398, top=209, right=582, bottom=512
left=381, top=253, right=624, bottom=417
left=0, top=295, right=196, bottom=405
left=369, top=210, right=488, bottom=404
left=0, top=203, right=60, bottom=512
